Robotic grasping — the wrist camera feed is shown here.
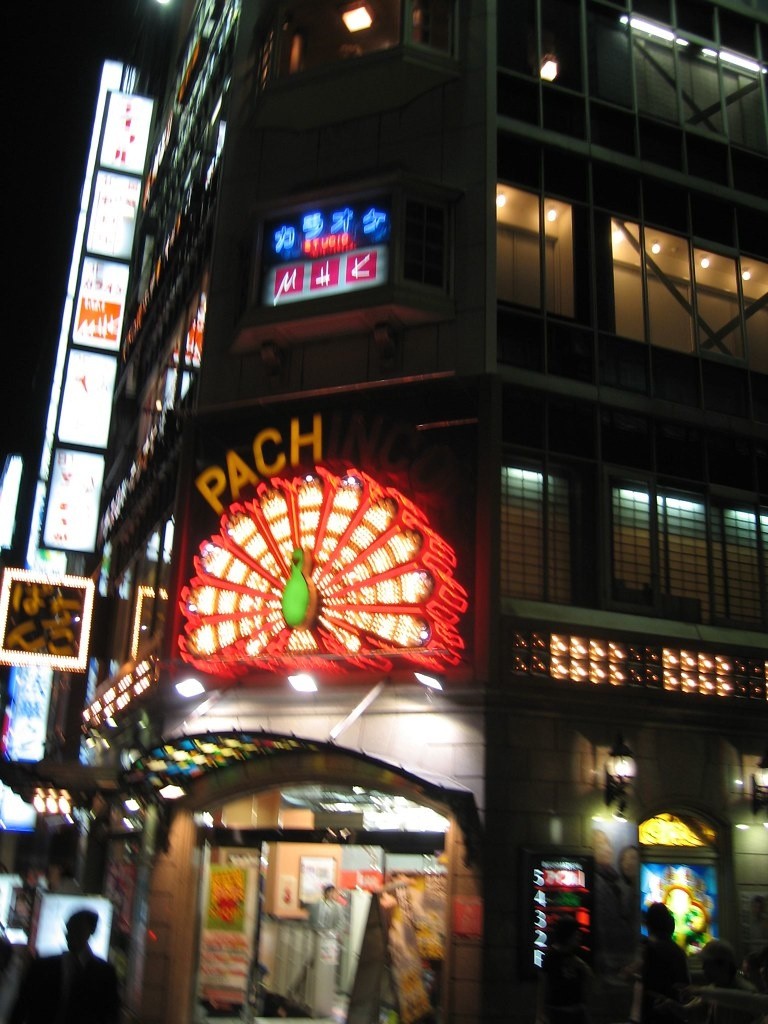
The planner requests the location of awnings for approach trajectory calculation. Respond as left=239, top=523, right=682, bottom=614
left=111, top=721, right=480, bottom=854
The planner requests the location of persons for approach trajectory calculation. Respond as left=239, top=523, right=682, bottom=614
left=652, top=940, right=768, bottom=1024
left=740, top=895, right=768, bottom=994
left=0, top=910, right=122, bottom=1024
left=589, top=822, right=641, bottom=907
left=290, top=886, right=348, bottom=998
left=622, top=904, right=689, bottom=1024
left=543, top=919, right=604, bottom=1024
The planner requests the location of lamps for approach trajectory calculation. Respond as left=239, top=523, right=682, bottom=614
left=413, top=671, right=447, bottom=703
left=540, top=54, right=558, bottom=82
left=749, top=754, right=768, bottom=816
left=619, top=11, right=768, bottom=81
left=602, top=729, right=639, bottom=819
left=287, top=671, right=318, bottom=693
left=172, top=673, right=207, bottom=699
